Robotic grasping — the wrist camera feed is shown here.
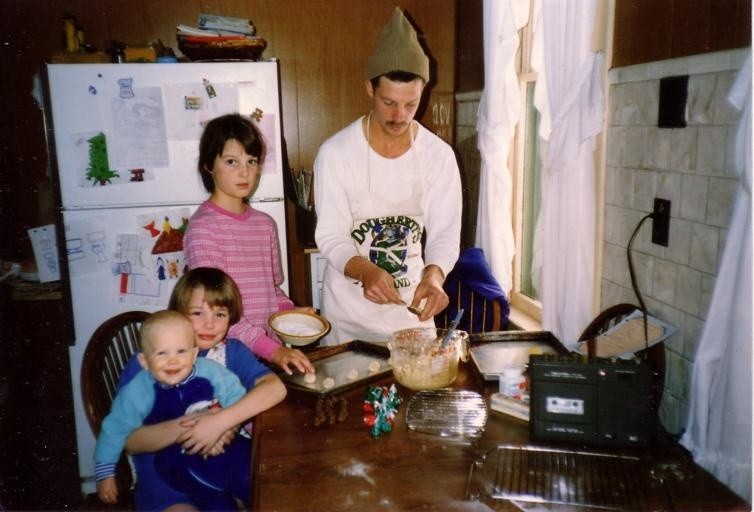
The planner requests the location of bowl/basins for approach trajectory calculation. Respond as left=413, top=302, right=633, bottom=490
left=176, top=36, right=268, bottom=59
left=386, top=328, right=462, bottom=391
left=269, top=311, right=331, bottom=350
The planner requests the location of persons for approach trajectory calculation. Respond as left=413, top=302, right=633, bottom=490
left=313, top=71, right=465, bottom=347
left=183, top=112, right=317, bottom=379
left=114, top=267, right=289, bottom=462
left=93, top=310, right=254, bottom=511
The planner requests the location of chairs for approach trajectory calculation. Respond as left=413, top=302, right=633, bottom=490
left=78, top=310, right=156, bottom=511
left=433, top=269, right=501, bottom=332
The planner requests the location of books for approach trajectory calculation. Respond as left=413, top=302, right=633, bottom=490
left=490, top=391, right=532, bottom=413
left=490, top=403, right=531, bottom=426
left=176, top=12, right=266, bottom=51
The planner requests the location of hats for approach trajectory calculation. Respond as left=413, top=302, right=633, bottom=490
left=367, top=5, right=431, bottom=85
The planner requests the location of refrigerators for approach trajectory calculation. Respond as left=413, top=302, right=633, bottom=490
left=43, top=60, right=291, bottom=495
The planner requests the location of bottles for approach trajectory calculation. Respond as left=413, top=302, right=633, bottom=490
left=497, top=364, right=531, bottom=398
left=63, top=14, right=85, bottom=52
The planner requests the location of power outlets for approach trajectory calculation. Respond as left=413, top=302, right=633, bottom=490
left=649, top=195, right=673, bottom=248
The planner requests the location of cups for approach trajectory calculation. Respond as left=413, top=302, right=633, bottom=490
left=454, top=330, right=470, bottom=365
